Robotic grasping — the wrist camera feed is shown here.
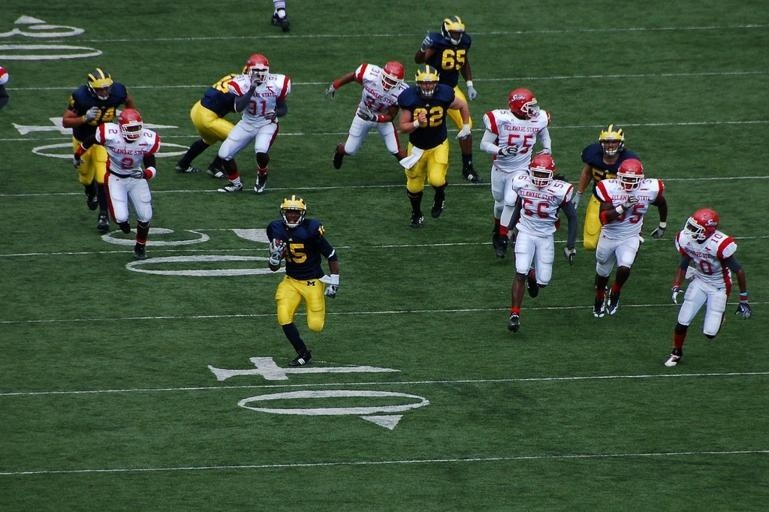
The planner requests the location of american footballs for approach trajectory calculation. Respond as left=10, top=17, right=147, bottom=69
left=272, top=239, right=286, bottom=261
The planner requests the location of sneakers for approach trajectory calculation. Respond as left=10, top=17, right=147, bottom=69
left=95, top=211, right=111, bottom=232
left=410, top=210, right=426, bottom=230
left=133, top=245, right=149, bottom=259
left=492, top=239, right=507, bottom=260
left=119, top=222, right=132, bottom=234
left=505, top=312, right=521, bottom=334
left=607, top=289, right=620, bottom=317
left=429, top=194, right=445, bottom=220
left=204, top=164, right=228, bottom=181
left=85, top=195, right=98, bottom=212
left=332, top=144, right=346, bottom=171
left=461, top=168, right=481, bottom=182
left=290, top=351, right=314, bottom=365
left=526, top=266, right=540, bottom=298
left=594, top=295, right=607, bottom=320
left=253, top=171, right=268, bottom=193
left=270, top=14, right=290, bottom=28
left=175, top=161, right=199, bottom=174
left=216, top=182, right=245, bottom=195
left=663, top=352, right=684, bottom=368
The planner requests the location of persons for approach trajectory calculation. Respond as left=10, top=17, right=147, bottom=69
left=480, top=88, right=552, bottom=257
left=324, top=63, right=410, bottom=171
left=665, top=209, right=751, bottom=367
left=216, top=54, right=291, bottom=195
left=414, top=15, right=478, bottom=181
left=271, top=0, right=291, bottom=32
left=398, top=66, right=471, bottom=227
left=72, top=108, right=161, bottom=260
left=174, top=63, right=249, bottom=179
left=593, top=158, right=668, bottom=318
left=577, top=123, right=640, bottom=250
left=266, top=196, right=340, bottom=366
left=62, top=68, right=134, bottom=232
left=502, top=155, right=579, bottom=330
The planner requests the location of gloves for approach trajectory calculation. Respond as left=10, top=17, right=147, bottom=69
left=497, top=145, right=518, bottom=160
left=735, top=302, right=753, bottom=321
left=455, top=124, right=472, bottom=140
left=264, top=110, right=279, bottom=123
left=251, top=78, right=264, bottom=89
left=131, top=169, right=146, bottom=181
left=267, top=238, right=286, bottom=266
left=71, top=157, right=87, bottom=169
left=82, top=106, right=100, bottom=123
left=670, top=284, right=685, bottom=304
left=564, top=247, right=576, bottom=265
left=356, top=105, right=376, bottom=123
left=649, top=225, right=667, bottom=240
left=570, top=190, right=583, bottom=210
left=621, top=196, right=639, bottom=214
left=466, top=86, right=479, bottom=101
left=504, top=231, right=516, bottom=248
left=323, top=284, right=340, bottom=299
left=325, top=83, right=337, bottom=100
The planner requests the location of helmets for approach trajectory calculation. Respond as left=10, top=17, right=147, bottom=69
left=414, top=64, right=441, bottom=98
left=278, top=194, right=309, bottom=229
left=527, top=153, right=556, bottom=189
left=507, top=88, right=542, bottom=119
left=683, top=209, right=720, bottom=242
left=441, top=16, right=466, bottom=47
left=380, top=62, right=406, bottom=93
left=87, top=64, right=112, bottom=102
left=617, top=158, right=646, bottom=193
left=118, top=108, right=143, bottom=142
left=599, top=123, right=626, bottom=158
left=246, top=52, right=270, bottom=86
